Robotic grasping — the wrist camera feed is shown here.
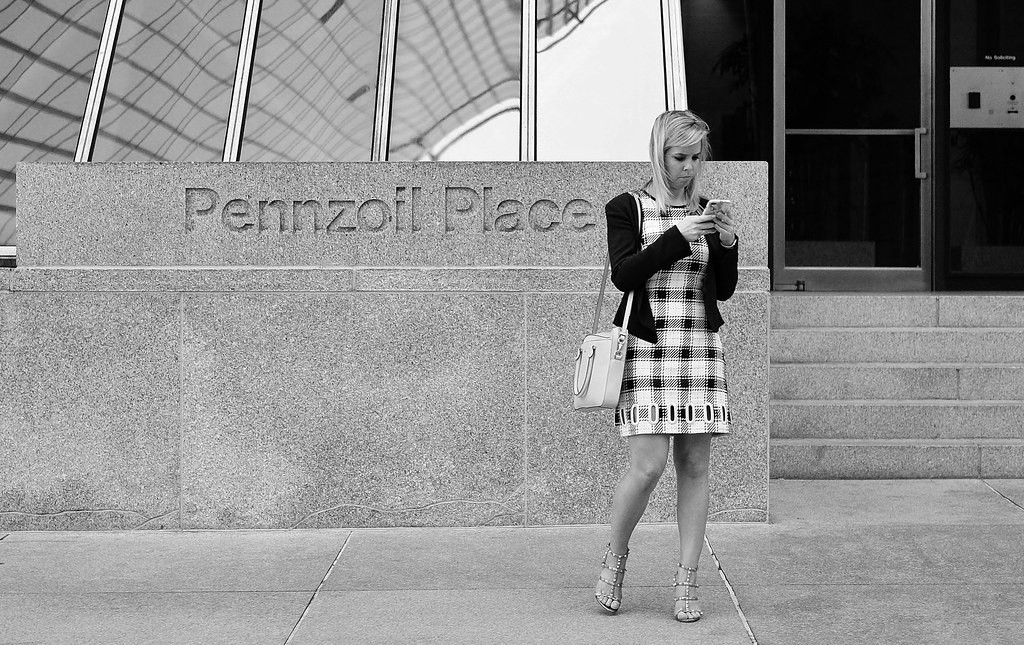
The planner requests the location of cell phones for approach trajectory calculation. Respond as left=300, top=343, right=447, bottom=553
left=701, top=199, right=730, bottom=229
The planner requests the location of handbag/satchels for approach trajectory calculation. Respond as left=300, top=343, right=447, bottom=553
left=572, top=327, right=628, bottom=412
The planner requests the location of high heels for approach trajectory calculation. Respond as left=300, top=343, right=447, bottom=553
left=595, top=541, right=629, bottom=613
left=673, top=562, right=703, bottom=623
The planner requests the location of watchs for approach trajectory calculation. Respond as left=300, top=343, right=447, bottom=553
left=720, top=239, right=737, bottom=249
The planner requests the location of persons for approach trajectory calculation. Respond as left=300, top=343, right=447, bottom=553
left=593, top=108, right=739, bottom=623
